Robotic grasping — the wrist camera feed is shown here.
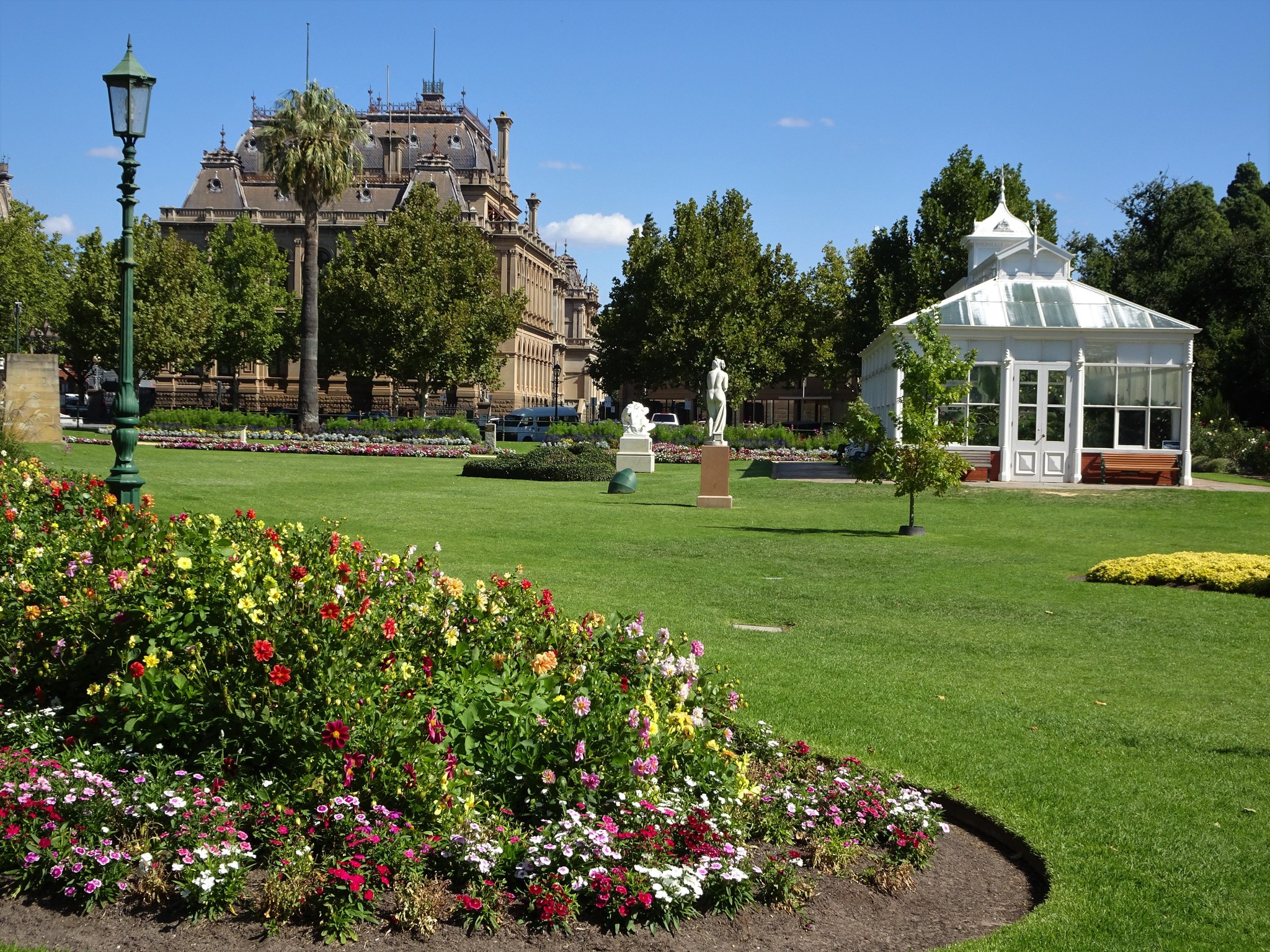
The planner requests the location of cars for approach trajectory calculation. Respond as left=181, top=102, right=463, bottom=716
left=334, top=409, right=392, bottom=423
left=650, top=412, right=680, bottom=426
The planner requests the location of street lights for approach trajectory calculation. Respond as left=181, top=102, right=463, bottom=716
left=100, top=33, right=160, bottom=520
left=553, top=359, right=562, bottom=425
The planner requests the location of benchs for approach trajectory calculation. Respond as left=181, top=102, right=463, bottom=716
left=842, top=440, right=880, bottom=471
left=1099, top=452, right=1183, bottom=487
left=945, top=449, right=994, bottom=484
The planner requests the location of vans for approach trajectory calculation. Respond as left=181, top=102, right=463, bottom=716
left=496, top=406, right=581, bottom=442
